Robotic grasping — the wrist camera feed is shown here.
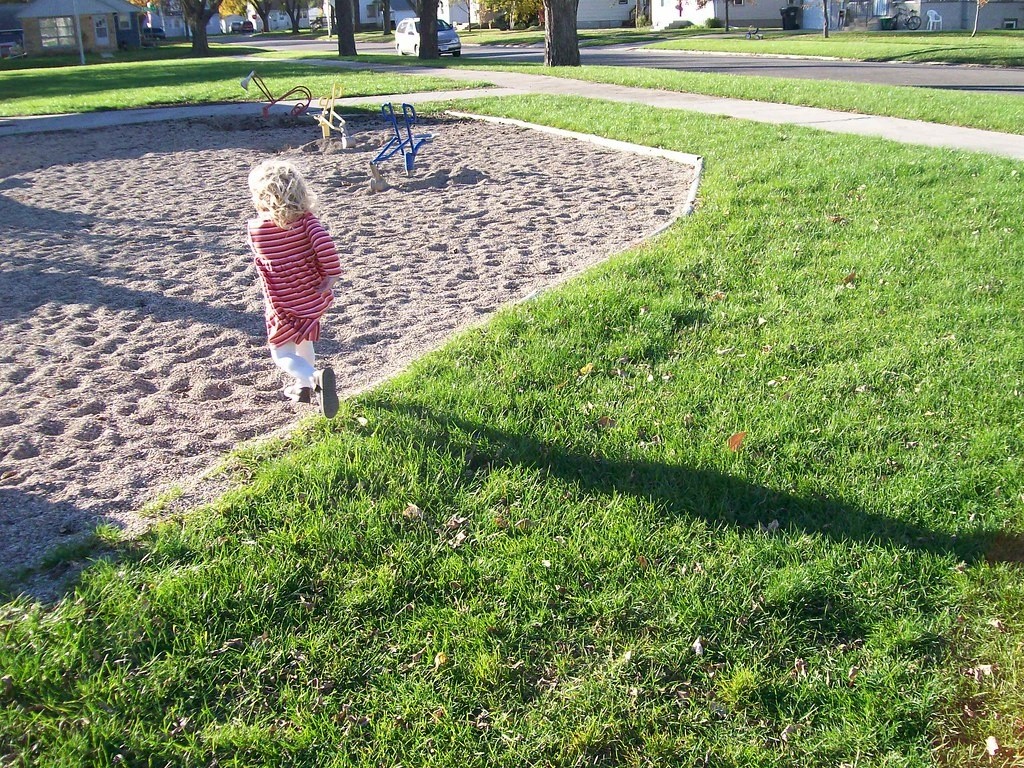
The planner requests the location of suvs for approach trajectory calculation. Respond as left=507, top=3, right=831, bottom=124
left=144, top=27, right=167, bottom=41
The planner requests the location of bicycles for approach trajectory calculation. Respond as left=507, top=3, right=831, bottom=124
left=888, top=1, right=922, bottom=31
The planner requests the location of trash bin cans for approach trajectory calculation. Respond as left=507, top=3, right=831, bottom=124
left=779, top=5, right=799, bottom=30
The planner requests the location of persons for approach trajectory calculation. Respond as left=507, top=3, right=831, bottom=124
left=245, top=160, right=344, bottom=418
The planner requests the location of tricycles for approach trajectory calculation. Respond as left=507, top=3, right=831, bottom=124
left=745, top=24, right=763, bottom=40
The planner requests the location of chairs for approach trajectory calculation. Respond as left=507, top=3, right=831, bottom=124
left=926, top=9, right=942, bottom=31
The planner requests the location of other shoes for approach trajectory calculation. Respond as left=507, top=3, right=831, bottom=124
left=312, top=367, right=339, bottom=418
left=283, top=385, right=310, bottom=402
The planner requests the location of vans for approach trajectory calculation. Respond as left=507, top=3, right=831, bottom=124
left=394, top=16, right=462, bottom=58
left=230, top=20, right=254, bottom=33
